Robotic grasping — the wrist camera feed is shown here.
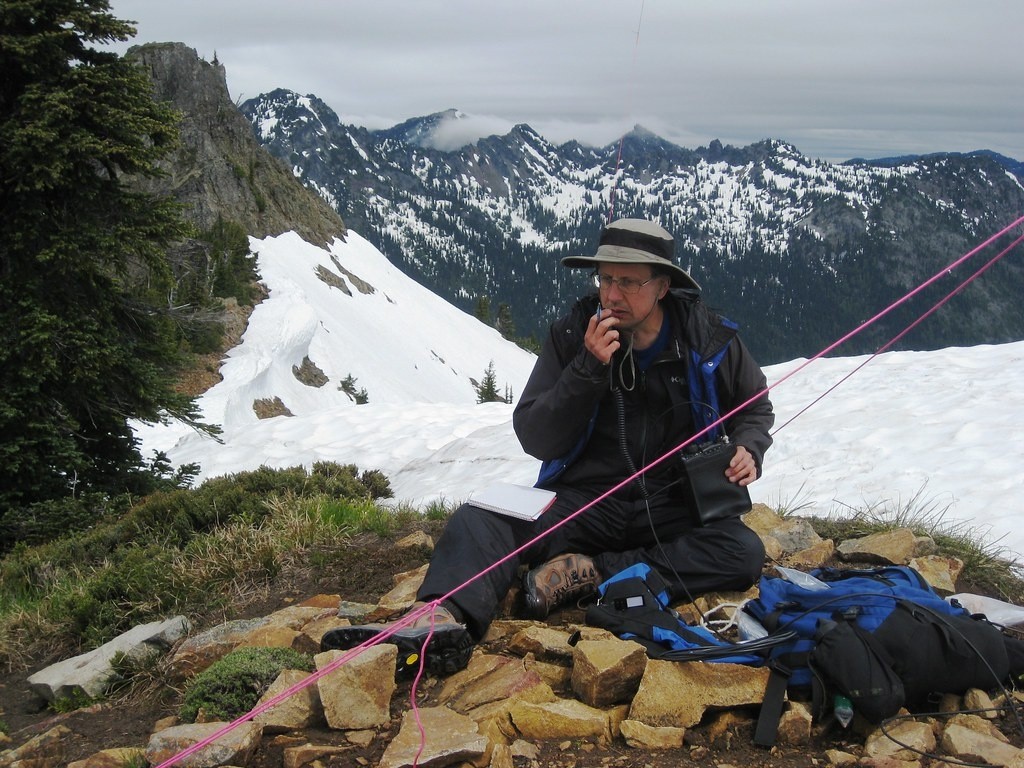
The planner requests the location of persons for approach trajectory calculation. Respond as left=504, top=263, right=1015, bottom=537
left=317, top=216, right=777, bottom=684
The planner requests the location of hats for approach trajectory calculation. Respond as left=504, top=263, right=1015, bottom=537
left=560, top=218, right=703, bottom=292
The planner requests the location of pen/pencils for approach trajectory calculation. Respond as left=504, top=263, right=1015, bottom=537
left=597, top=303, right=601, bottom=323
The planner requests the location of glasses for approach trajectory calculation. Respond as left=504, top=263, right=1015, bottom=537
left=589, top=269, right=658, bottom=292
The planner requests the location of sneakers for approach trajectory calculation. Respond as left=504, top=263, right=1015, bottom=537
left=319, top=602, right=475, bottom=681
left=517, top=554, right=603, bottom=618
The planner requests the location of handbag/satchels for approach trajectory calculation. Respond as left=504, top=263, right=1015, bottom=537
left=874, top=601, right=1010, bottom=705
left=809, top=616, right=907, bottom=729
